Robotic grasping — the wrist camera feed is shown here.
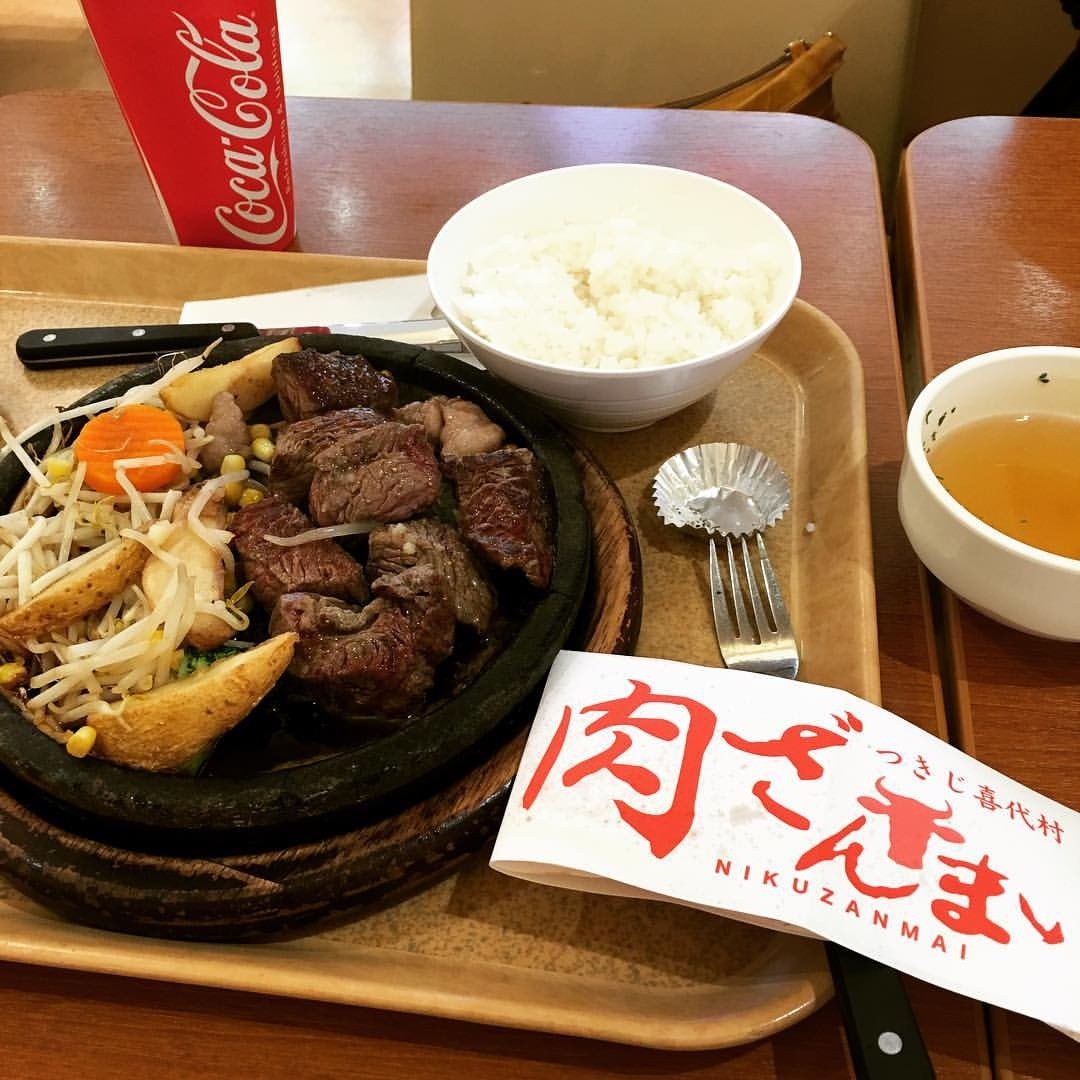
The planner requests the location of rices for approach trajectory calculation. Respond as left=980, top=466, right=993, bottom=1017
left=457, top=192, right=776, bottom=371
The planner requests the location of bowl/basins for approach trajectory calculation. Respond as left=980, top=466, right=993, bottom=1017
left=895, top=345, right=1080, bottom=639
left=426, top=160, right=801, bottom=435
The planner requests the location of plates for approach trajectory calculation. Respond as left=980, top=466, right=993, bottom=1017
left=1, top=332, right=647, bottom=936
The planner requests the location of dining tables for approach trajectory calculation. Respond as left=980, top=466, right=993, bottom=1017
left=896, top=113, right=1080, bottom=812
left=1, top=88, right=993, bottom=1080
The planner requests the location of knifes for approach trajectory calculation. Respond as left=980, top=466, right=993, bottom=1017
left=16, top=317, right=472, bottom=371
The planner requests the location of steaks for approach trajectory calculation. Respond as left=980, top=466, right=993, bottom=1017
left=233, top=336, right=549, bottom=717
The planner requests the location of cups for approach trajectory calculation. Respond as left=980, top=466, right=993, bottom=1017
left=78, top=0, right=299, bottom=252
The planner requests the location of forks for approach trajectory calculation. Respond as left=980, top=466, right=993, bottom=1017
left=703, top=532, right=939, bottom=1080
